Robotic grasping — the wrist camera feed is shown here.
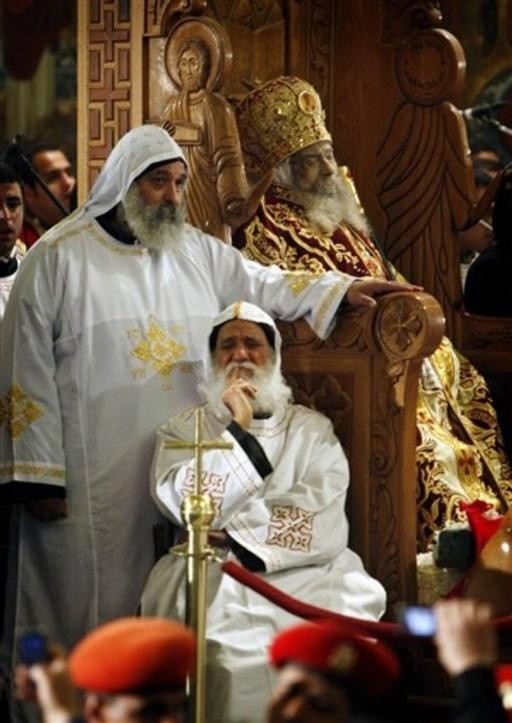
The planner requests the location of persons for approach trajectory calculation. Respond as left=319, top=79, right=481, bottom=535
left=14, top=646, right=88, bottom=723
left=157, top=40, right=249, bottom=248
left=0, top=157, right=29, bottom=311
left=0, top=124, right=427, bottom=723
left=20, top=136, right=78, bottom=248
left=266, top=621, right=402, bottom=723
left=67, top=617, right=197, bottom=723
left=136, top=299, right=389, bottom=720
left=436, top=1, right=511, bottom=321
left=432, top=600, right=507, bottom=723
left=245, top=68, right=512, bottom=539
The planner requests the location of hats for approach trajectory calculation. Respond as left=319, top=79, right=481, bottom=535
left=66, top=614, right=196, bottom=692
left=235, top=74, right=332, bottom=175
left=270, top=617, right=402, bottom=698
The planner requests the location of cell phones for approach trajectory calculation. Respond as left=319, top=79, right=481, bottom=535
left=16, top=632, right=48, bottom=667
left=404, top=604, right=438, bottom=636
